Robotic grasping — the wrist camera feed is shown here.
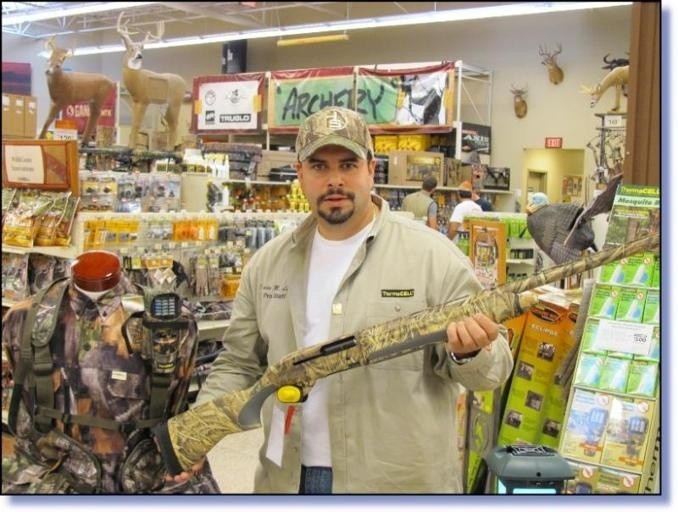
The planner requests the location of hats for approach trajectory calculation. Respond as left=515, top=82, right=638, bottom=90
left=295, top=105, right=373, bottom=161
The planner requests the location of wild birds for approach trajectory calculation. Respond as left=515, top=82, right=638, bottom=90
left=527, top=172, right=622, bottom=287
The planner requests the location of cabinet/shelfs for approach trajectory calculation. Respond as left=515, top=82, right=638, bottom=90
left=4, top=93, right=313, bottom=404
left=369, top=62, right=514, bottom=236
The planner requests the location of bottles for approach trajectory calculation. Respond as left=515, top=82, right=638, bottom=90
left=84, top=185, right=310, bottom=249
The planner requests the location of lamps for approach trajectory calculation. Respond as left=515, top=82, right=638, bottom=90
left=39, top=2, right=634, bottom=57
left=1, top=1, right=150, bottom=28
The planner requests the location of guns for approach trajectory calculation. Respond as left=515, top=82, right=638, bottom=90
left=156, top=235, right=660, bottom=478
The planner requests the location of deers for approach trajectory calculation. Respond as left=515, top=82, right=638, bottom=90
left=537, top=42, right=565, bottom=86
left=115, top=10, right=187, bottom=153
left=37, top=35, right=114, bottom=150
left=506, top=81, right=530, bottom=119
left=577, top=49, right=630, bottom=111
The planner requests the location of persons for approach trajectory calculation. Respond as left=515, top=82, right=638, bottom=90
left=2, top=251, right=199, bottom=494
left=447, top=181, right=496, bottom=240
left=154, top=106, right=513, bottom=495
left=401, top=176, right=440, bottom=230
